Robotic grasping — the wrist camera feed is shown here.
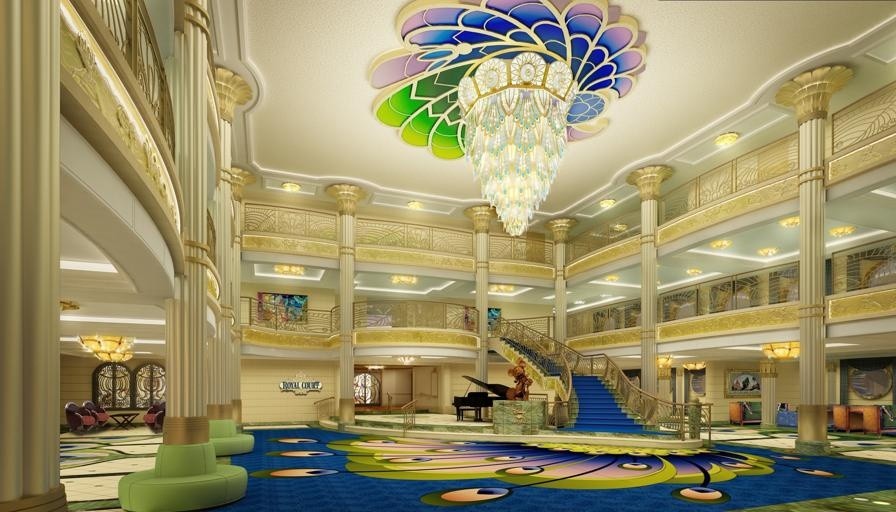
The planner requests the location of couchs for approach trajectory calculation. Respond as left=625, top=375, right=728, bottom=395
left=729, top=401, right=896, bottom=436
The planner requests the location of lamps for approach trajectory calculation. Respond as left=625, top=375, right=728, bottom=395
left=781, top=216, right=801, bottom=229
left=762, top=342, right=802, bottom=359
left=78, top=334, right=134, bottom=362
left=682, top=361, right=706, bottom=370
left=60, top=301, right=79, bottom=312
left=601, top=199, right=616, bottom=211
left=709, top=240, right=731, bottom=250
left=715, top=133, right=739, bottom=147
left=757, top=247, right=778, bottom=256
left=830, top=226, right=855, bottom=238
left=613, top=225, right=627, bottom=232
left=606, top=276, right=617, bottom=282
left=656, top=355, right=672, bottom=369
left=271, top=183, right=424, bottom=285
left=686, top=270, right=701, bottom=276
left=490, top=284, right=514, bottom=293
left=456, top=51, right=578, bottom=237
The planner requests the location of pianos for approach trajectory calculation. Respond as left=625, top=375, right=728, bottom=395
left=452, top=376, right=511, bottom=421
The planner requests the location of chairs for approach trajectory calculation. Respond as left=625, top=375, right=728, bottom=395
left=65, top=402, right=95, bottom=432
left=143, top=402, right=165, bottom=429
left=83, top=400, right=109, bottom=426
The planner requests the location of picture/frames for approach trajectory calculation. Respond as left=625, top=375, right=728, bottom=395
left=724, top=368, right=762, bottom=398
